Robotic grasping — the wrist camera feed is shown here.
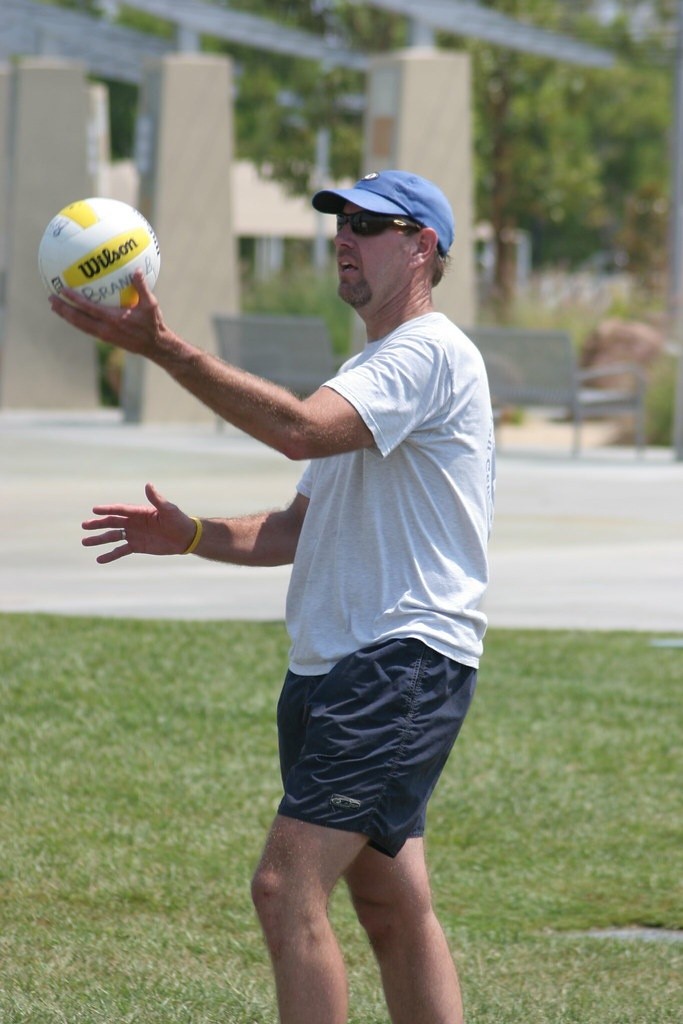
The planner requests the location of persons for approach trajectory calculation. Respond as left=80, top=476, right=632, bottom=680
left=81, top=171, right=495, bottom=1024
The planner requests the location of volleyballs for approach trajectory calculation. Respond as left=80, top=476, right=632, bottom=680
left=37, top=196, right=162, bottom=310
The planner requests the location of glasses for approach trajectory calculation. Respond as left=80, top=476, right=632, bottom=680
left=337, top=212, right=442, bottom=254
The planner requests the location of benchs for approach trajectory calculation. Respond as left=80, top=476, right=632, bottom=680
left=211, top=316, right=341, bottom=432
left=461, top=328, right=645, bottom=456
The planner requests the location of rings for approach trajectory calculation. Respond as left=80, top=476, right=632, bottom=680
left=121, top=530, right=126, bottom=540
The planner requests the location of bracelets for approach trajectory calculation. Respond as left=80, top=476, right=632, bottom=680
left=182, top=517, right=203, bottom=555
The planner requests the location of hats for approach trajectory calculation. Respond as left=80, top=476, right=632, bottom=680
left=312, top=169, right=456, bottom=258
